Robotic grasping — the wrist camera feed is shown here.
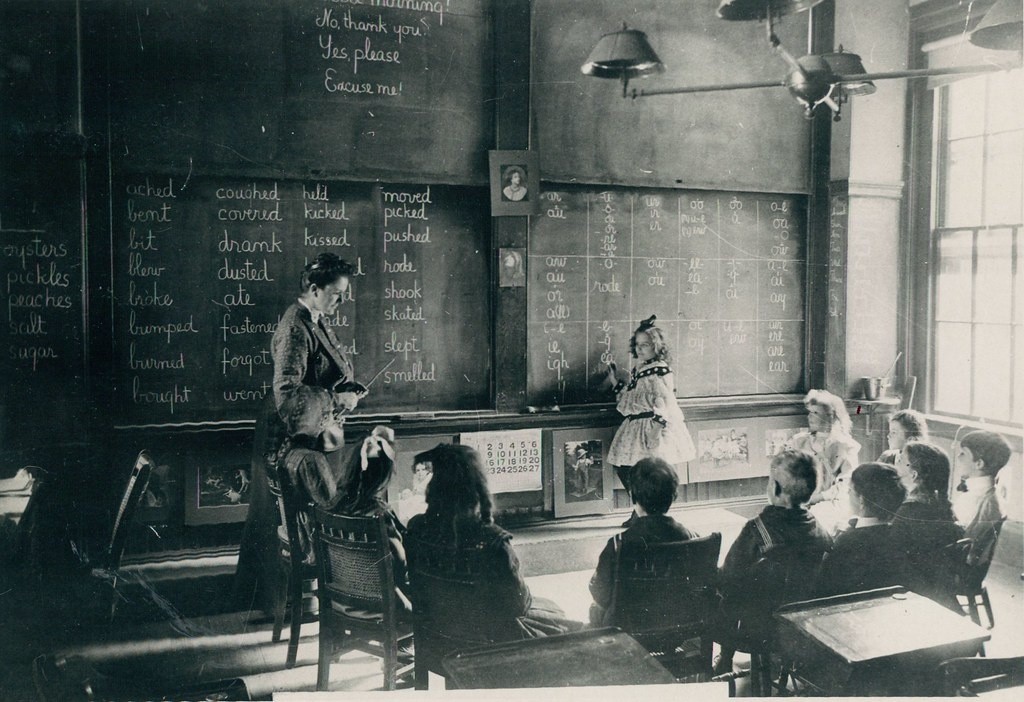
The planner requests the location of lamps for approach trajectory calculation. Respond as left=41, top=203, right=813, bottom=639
left=579, top=1, right=1024, bottom=123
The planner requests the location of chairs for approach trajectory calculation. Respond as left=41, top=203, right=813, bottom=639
left=401, top=531, right=522, bottom=690
left=307, top=502, right=415, bottom=691
left=38, top=449, right=156, bottom=624
left=54, top=654, right=251, bottom=702
left=610, top=533, right=721, bottom=683
left=263, top=463, right=318, bottom=669
left=933, top=658, right=1024, bottom=697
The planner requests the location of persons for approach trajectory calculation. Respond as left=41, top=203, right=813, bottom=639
left=876, top=407, right=929, bottom=464
left=325, top=434, right=415, bottom=689
left=571, top=449, right=594, bottom=495
left=585, top=458, right=701, bottom=631
left=229, top=250, right=368, bottom=612
left=710, top=447, right=838, bottom=677
left=950, top=430, right=1012, bottom=617
left=607, top=314, right=697, bottom=530
left=782, top=388, right=861, bottom=539
left=406, top=443, right=585, bottom=656
left=399, top=452, right=434, bottom=523
left=811, top=461, right=913, bottom=600
left=269, top=382, right=338, bottom=568
left=889, top=439, right=964, bottom=587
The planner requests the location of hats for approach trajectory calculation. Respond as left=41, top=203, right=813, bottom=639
left=578, top=449, right=588, bottom=459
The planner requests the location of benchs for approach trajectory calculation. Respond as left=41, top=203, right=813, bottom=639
left=708, top=515, right=1007, bottom=698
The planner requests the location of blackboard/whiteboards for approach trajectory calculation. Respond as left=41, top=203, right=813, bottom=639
left=0, top=160, right=93, bottom=404
left=0, top=1, right=85, bottom=137
left=115, top=175, right=494, bottom=415
left=530, top=0, right=812, bottom=174
left=112, top=0, right=494, bottom=171
left=526, top=179, right=810, bottom=405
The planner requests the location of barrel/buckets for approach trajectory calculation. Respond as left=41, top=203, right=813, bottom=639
left=859, top=376, right=892, bottom=401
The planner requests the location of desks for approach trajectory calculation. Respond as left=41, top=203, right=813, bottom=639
left=774, top=585, right=993, bottom=697
left=441, top=626, right=677, bottom=690
left=0, top=478, right=40, bottom=552
